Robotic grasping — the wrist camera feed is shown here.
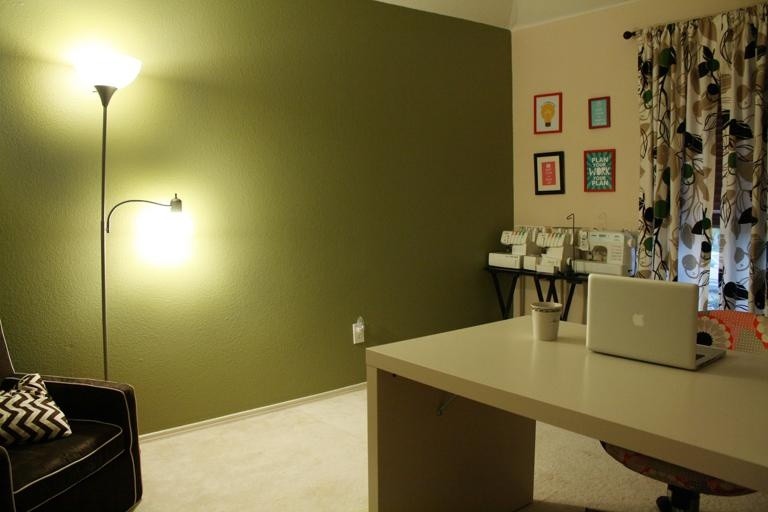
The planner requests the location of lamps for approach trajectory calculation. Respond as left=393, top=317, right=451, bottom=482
left=67, top=44, right=195, bottom=381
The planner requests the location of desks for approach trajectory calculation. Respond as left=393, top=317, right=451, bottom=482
left=365, top=314, right=768, bottom=512
left=547, top=272, right=589, bottom=321
left=484, top=266, right=556, bottom=320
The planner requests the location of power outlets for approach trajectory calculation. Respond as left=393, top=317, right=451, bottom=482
left=352, top=323, right=365, bottom=345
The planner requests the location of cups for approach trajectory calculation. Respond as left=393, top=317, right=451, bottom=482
left=529, top=301, right=562, bottom=343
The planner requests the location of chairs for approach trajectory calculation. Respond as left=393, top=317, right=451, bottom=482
left=600, top=309, right=768, bottom=511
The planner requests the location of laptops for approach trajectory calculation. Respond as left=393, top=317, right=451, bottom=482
left=585, top=272, right=727, bottom=370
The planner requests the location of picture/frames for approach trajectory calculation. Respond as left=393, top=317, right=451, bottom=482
left=533, top=92, right=563, bottom=135
left=587, top=96, right=611, bottom=129
left=533, top=151, right=565, bottom=195
left=583, top=149, right=616, bottom=193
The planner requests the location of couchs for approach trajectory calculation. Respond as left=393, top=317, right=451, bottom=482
left=0, top=316, right=142, bottom=512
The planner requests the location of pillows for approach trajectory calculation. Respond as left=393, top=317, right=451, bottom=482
left=0, top=373, right=72, bottom=445
